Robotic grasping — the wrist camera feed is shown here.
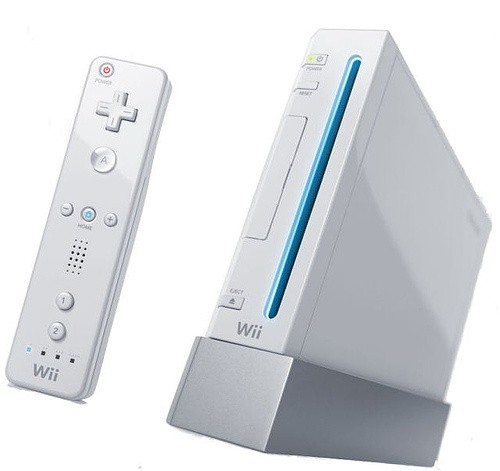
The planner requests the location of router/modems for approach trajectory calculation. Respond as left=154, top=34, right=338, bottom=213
left=165, top=28, right=493, bottom=470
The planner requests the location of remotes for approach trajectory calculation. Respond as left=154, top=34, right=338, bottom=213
left=5, top=55, right=173, bottom=403
